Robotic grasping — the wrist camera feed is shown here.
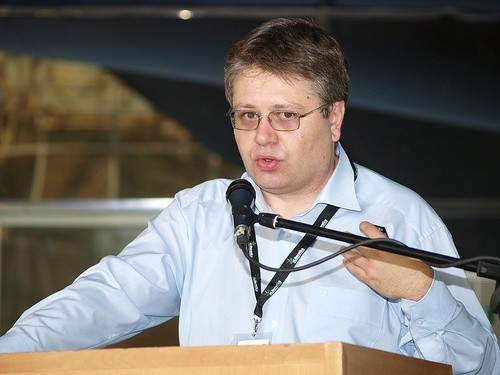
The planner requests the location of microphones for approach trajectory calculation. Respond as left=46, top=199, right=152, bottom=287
left=226, top=179, right=256, bottom=249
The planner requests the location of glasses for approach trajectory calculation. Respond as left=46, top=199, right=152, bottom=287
left=226, top=104, right=326, bottom=132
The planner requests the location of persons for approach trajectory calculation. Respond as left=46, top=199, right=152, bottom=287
left=1, top=16, right=500, bottom=375
